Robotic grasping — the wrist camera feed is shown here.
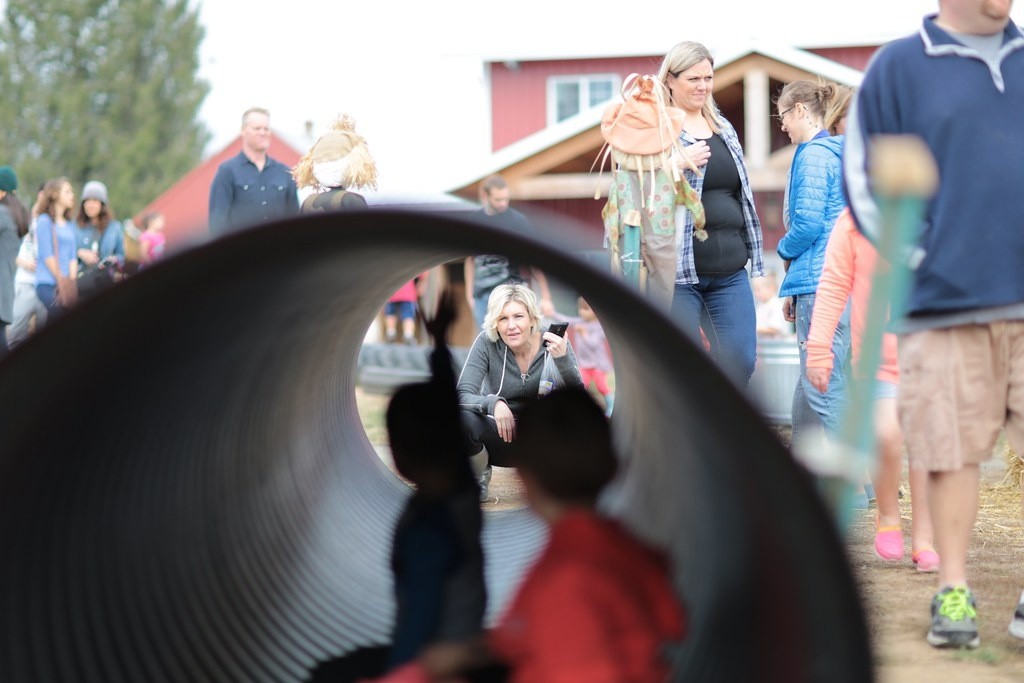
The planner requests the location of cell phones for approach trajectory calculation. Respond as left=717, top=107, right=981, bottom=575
left=542, top=322, right=570, bottom=347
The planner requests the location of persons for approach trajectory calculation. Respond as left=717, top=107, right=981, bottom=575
left=291, top=113, right=378, bottom=216
left=371, top=382, right=688, bottom=683
left=642, top=41, right=766, bottom=389
left=386, top=288, right=487, bottom=669
left=457, top=283, right=586, bottom=503
left=599, top=72, right=706, bottom=311
left=382, top=271, right=429, bottom=343
left=777, top=79, right=881, bottom=509
left=206, top=107, right=300, bottom=239
left=839, top=0, right=1024, bottom=649
left=464, top=175, right=552, bottom=329
left=0, top=166, right=166, bottom=352
left=566, top=294, right=615, bottom=416
left=807, top=206, right=940, bottom=574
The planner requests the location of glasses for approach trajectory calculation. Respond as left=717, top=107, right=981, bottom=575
left=778, top=103, right=808, bottom=123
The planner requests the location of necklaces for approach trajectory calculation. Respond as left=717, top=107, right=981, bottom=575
left=522, top=344, right=532, bottom=381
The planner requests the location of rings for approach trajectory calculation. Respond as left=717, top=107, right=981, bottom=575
left=546, top=342, right=550, bottom=346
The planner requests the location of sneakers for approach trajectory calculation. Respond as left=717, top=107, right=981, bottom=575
left=874, top=513, right=905, bottom=562
left=925, top=584, right=980, bottom=649
left=1009, top=589, right=1024, bottom=640
left=911, top=548, right=939, bottom=573
left=476, top=468, right=493, bottom=502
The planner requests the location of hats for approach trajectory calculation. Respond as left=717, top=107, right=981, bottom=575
left=0, top=166, right=17, bottom=191
left=82, top=181, right=108, bottom=204
